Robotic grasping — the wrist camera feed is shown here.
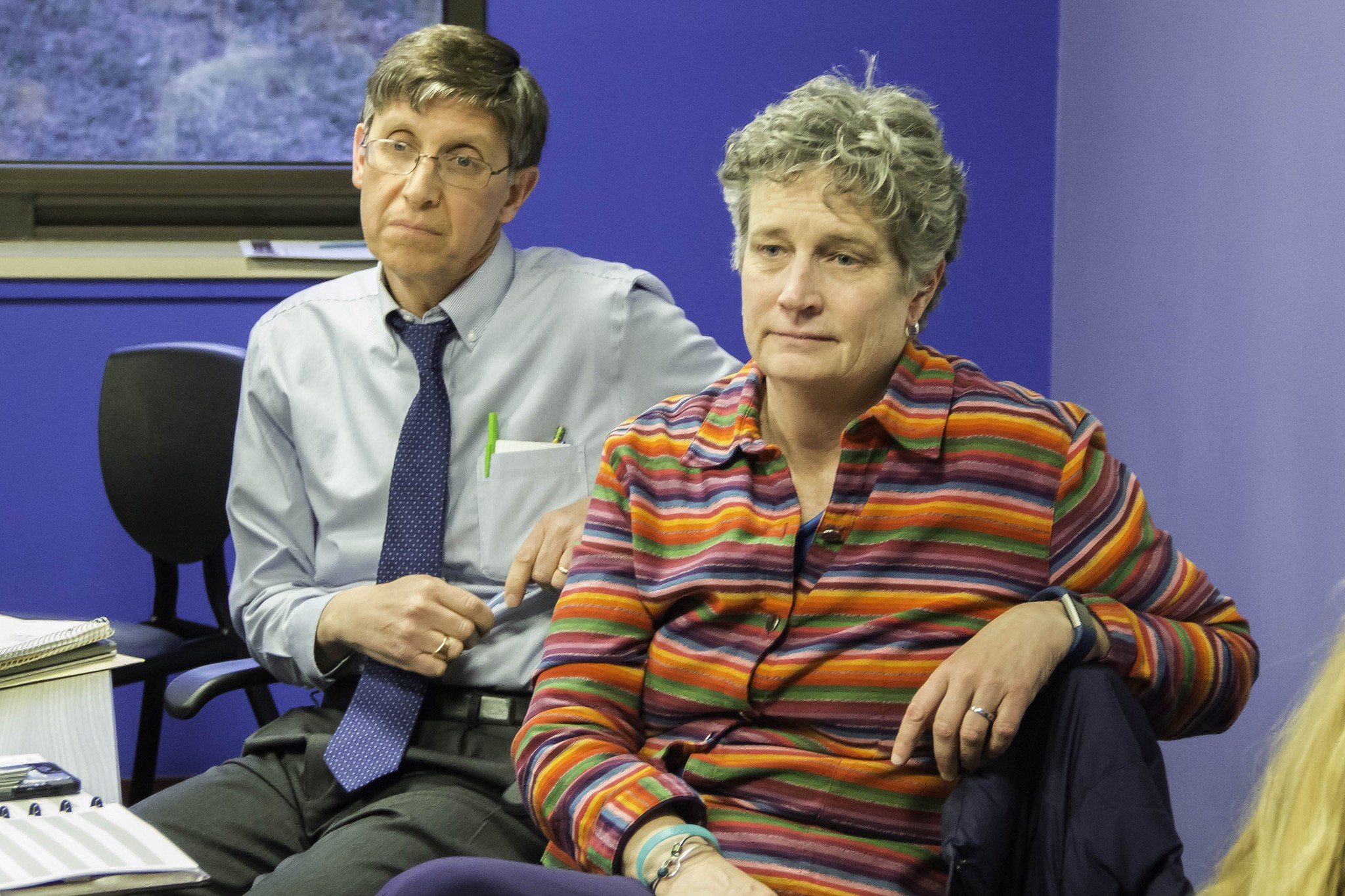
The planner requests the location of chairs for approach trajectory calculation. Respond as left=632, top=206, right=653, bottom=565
left=0, top=339, right=1194, bottom=895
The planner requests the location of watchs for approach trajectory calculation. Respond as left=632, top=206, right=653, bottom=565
left=1028, top=586, right=1098, bottom=670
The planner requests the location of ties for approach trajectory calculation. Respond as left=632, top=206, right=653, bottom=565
left=324, top=310, right=459, bottom=796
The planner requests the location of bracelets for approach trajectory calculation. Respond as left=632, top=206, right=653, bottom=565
left=636, top=822, right=720, bottom=883
left=648, top=834, right=716, bottom=894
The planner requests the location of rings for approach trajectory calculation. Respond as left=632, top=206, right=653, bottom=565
left=970, top=706, right=993, bottom=721
left=557, top=566, right=569, bottom=574
left=433, top=636, right=448, bottom=660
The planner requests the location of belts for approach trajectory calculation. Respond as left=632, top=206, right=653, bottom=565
left=320, top=678, right=530, bottom=725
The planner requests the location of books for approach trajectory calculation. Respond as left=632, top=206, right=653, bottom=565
left=0, top=799, right=215, bottom=896
left=0, top=613, right=118, bottom=682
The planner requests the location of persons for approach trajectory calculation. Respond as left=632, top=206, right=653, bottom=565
left=378, top=49, right=1261, bottom=896
left=126, top=25, right=746, bottom=896
left=1196, top=628, right=1345, bottom=896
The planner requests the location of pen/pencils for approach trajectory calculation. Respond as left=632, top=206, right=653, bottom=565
left=552, top=426, right=566, bottom=443
left=485, top=411, right=499, bottom=479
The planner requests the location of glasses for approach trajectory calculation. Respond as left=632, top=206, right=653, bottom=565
left=359, top=121, right=516, bottom=192
